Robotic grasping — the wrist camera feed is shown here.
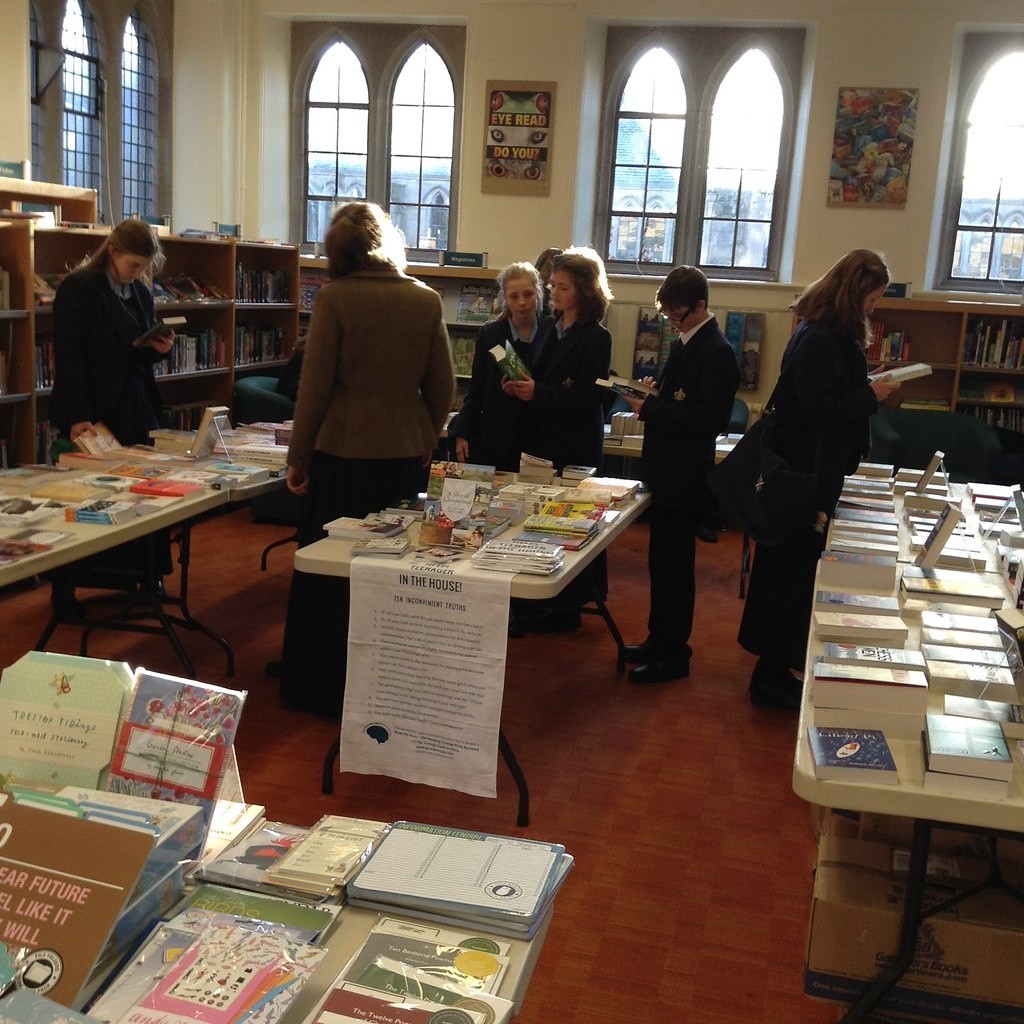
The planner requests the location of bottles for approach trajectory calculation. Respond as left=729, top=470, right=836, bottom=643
left=903, top=337, right=912, bottom=361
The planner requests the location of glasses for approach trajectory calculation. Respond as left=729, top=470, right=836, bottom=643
left=658, top=303, right=696, bottom=324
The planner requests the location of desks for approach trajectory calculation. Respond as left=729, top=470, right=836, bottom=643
left=794, top=554, right=1024, bottom=1024
left=53, top=462, right=301, bottom=677
left=277, top=900, right=560, bottom=1024
left=0, top=465, right=229, bottom=685
left=293, top=475, right=653, bottom=827
left=825, top=479, right=1024, bottom=574
left=441, top=421, right=754, bottom=600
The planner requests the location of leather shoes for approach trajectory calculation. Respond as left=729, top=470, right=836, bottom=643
left=623, top=644, right=647, bottom=662
left=628, top=657, right=689, bottom=681
left=749, top=658, right=803, bottom=711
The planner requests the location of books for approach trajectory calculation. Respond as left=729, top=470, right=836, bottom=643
left=806, top=462, right=1024, bottom=783
left=488, top=340, right=530, bottom=382
left=0, top=785, right=574, bottom=1024
left=960, top=319, right=1024, bottom=370
left=322, top=452, right=646, bottom=576
left=866, top=363, right=933, bottom=382
left=0, top=401, right=293, bottom=565
left=429, top=284, right=504, bottom=376
left=863, top=319, right=911, bottom=361
left=604, top=412, right=643, bottom=447
left=300, top=276, right=331, bottom=349
left=34, top=272, right=67, bottom=388
left=133, top=316, right=186, bottom=348
left=962, top=405, right=1024, bottom=433
left=153, top=272, right=231, bottom=375
left=716, top=433, right=744, bottom=455
left=234, top=262, right=289, bottom=366
left=595, top=375, right=658, bottom=400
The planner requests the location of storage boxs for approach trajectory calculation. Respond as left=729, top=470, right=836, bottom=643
left=802, top=807, right=1024, bottom=1024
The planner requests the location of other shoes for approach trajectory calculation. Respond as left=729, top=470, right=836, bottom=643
left=141, top=578, right=167, bottom=598
left=51, top=586, right=85, bottom=617
left=266, top=663, right=284, bottom=679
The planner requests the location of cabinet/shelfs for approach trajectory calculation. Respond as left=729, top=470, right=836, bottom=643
left=0, top=175, right=299, bottom=468
left=300, top=254, right=503, bottom=377
left=792, top=292, right=1024, bottom=430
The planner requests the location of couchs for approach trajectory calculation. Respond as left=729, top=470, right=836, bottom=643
left=632, top=399, right=748, bottom=519
left=233, top=377, right=304, bottom=528
left=843, top=409, right=1001, bottom=484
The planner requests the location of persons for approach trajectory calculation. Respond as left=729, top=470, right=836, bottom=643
left=622, top=265, right=741, bottom=685
left=279, top=201, right=456, bottom=717
left=738, top=248, right=902, bottom=709
left=455, top=248, right=564, bottom=462
left=501, top=246, right=612, bottom=631
left=52, top=218, right=176, bottom=446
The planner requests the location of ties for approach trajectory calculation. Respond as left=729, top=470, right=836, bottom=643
left=659, top=339, right=683, bottom=390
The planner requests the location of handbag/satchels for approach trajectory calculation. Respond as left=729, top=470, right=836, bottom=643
left=705, top=411, right=822, bottom=552
